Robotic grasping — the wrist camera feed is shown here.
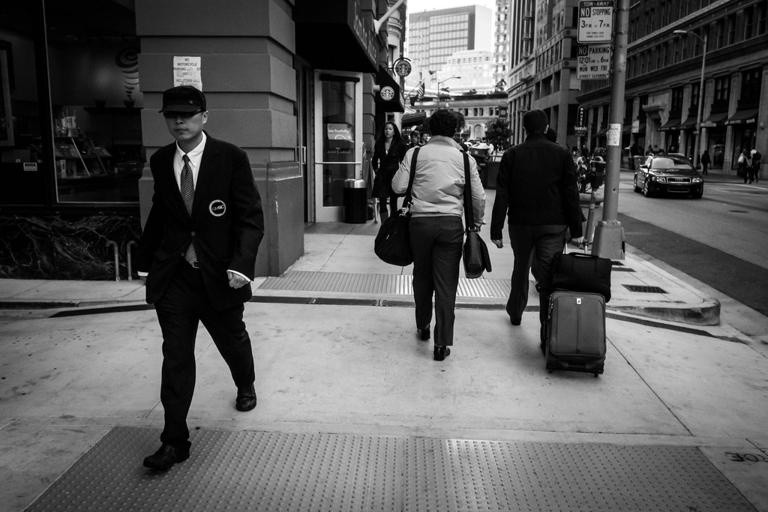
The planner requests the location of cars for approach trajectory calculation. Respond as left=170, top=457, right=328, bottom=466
left=711, top=143, right=724, bottom=166
left=633, top=154, right=705, bottom=200
left=575, top=155, right=605, bottom=179
left=464, top=141, right=474, bottom=150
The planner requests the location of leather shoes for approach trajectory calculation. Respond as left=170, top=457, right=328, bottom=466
left=416, top=325, right=429, bottom=340
left=143, top=441, right=191, bottom=473
left=434, top=346, right=449, bottom=361
left=236, top=383, right=255, bottom=412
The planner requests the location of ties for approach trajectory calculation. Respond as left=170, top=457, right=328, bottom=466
left=180, top=155, right=197, bottom=264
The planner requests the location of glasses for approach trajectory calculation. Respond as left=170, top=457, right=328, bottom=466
left=163, top=110, right=203, bottom=118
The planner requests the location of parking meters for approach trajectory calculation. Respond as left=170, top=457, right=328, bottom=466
left=579, top=155, right=605, bottom=250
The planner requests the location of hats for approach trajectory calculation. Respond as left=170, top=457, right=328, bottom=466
left=157, top=85, right=205, bottom=112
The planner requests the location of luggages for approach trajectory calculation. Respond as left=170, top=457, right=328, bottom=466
left=545, top=291, right=605, bottom=377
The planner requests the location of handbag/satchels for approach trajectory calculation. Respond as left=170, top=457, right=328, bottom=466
left=462, top=232, right=490, bottom=278
left=548, top=252, right=611, bottom=302
left=373, top=209, right=413, bottom=266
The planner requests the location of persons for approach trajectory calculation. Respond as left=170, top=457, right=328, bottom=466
left=453, top=134, right=504, bottom=155
left=491, top=108, right=586, bottom=325
left=137, top=84, right=266, bottom=469
left=372, top=122, right=431, bottom=224
left=544, top=128, right=760, bottom=185
left=391, top=108, right=487, bottom=361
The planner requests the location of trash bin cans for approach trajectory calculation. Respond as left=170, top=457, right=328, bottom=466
left=342, top=178, right=369, bottom=223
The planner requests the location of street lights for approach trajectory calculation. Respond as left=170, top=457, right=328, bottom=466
left=403, top=68, right=437, bottom=115
left=437, top=76, right=461, bottom=111
left=671, top=29, right=709, bottom=170
